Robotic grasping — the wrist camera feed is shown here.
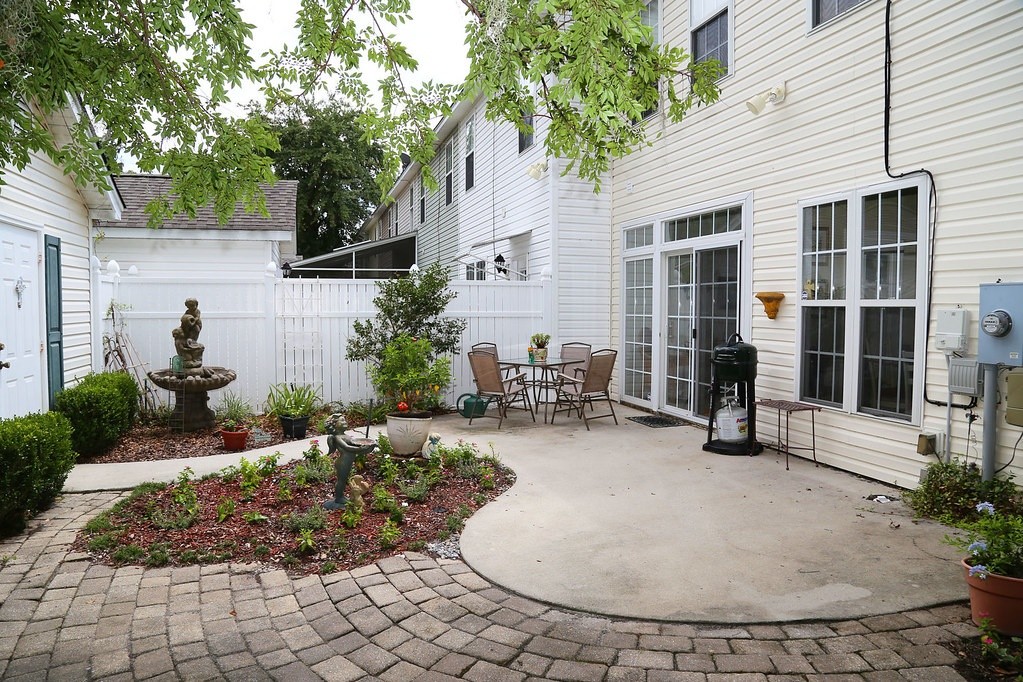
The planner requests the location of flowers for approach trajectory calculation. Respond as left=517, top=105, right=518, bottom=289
left=937, top=500, right=1023, bottom=581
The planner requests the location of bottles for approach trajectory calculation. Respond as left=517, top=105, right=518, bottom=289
left=527, top=345, right=534, bottom=364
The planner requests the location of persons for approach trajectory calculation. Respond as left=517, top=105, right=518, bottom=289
left=324, top=414, right=375, bottom=503
left=180, top=297, right=201, bottom=342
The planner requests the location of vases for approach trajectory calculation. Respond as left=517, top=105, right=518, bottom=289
left=960, top=555, right=1023, bottom=637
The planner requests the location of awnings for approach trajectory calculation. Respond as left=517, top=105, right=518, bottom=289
left=452, top=230, right=534, bottom=280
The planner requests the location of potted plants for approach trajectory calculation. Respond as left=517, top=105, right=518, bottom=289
left=219, top=419, right=259, bottom=450
left=530, top=333, right=550, bottom=362
left=367, top=331, right=452, bottom=455
left=264, top=381, right=327, bottom=439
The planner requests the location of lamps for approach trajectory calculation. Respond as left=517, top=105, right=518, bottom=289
left=527, top=159, right=549, bottom=179
left=280, top=262, right=292, bottom=278
left=746, top=77, right=786, bottom=117
left=494, top=254, right=509, bottom=277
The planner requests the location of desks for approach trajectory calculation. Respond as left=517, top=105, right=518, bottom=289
left=751, top=399, right=822, bottom=470
left=497, top=357, right=585, bottom=425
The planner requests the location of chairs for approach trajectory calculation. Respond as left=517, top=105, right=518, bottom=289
left=467, top=342, right=618, bottom=431
left=902, top=351, right=914, bottom=415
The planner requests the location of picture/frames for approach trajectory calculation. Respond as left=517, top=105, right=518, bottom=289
left=812, top=227, right=829, bottom=265
left=811, top=279, right=830, bottom=318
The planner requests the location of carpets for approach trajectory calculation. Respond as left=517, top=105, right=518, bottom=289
left=624, top=415, right=689, bottom=429
